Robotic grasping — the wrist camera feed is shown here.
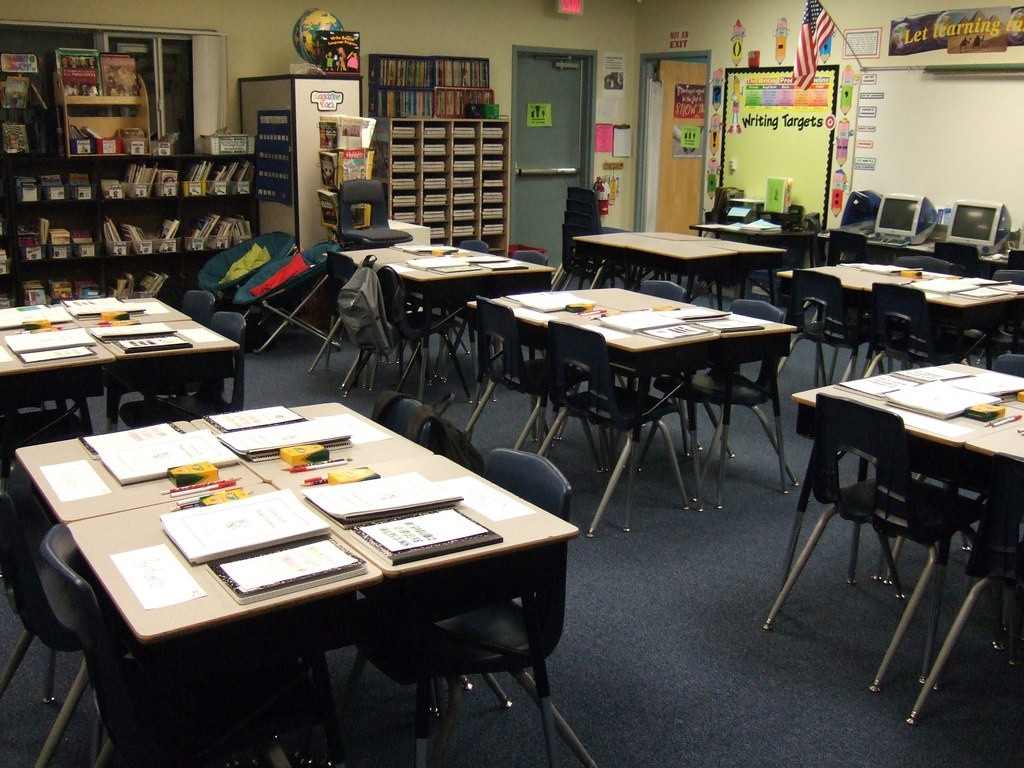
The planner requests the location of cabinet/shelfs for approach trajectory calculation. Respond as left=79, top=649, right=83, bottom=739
left=0, top=153, right=260, bottom=308
left=53, top=68, right=153, bottom=159
left=368, top=53, right=511, bottom=258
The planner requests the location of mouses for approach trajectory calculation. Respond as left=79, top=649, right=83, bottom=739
left=992, top=254, right=1008, bottom=260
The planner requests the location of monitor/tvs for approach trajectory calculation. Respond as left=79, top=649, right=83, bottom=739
left=841, top=190, right=939, bottom=245
left=945, top=201, right=1012, bottom=256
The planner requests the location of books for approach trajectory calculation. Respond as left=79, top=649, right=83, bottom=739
left=68, top=123, right=180, bottom=143
left=837, top=263, right=1024, bottom=300
left=0, top=75, right=30, bottom=155
left=392, top=125, right=504, bottom=237
left=78, top=404, right=504, bottom=604
left=379, top=57, right=492, bottom=117
left=316, top=114, right=377, bottom=233
left=0, top=299, right=193, bottom=362
left=54, top=45, right=139, bottom=98
left=17, top=159, right=254, bottom=258
left=834, top=367, right=1024, bottom=421
left=692, top=177, right=793, bottom=235
left=3, top=259, right=174, bottom=298
left=389, top=244, right=529, bottom=276
left=506, top=290, right=766, bottom=342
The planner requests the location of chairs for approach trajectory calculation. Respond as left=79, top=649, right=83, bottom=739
left=337, top=180, right=414, bottom=250
left=0, top=186, right=1024, bottom=767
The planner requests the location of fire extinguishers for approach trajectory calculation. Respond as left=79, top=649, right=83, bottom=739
left=592, top=177, right=610, bottom=215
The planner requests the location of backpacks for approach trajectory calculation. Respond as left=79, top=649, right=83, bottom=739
left=337, top=254, right=398, bottom=355
left=372, top=390, right=484, bottom=477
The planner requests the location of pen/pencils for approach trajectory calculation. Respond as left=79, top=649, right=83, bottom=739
left=298, top=478, right=328, bottom=487
left=281, top=454, right=357, bottom=474
left=468, top=258, right=511, bottom=263
left=94, top=321, right=115, bottom=327
left=897, top=280, right=916, bottom=286
left=575, top=309, right=607, bottom=318
left=161, top=478, right=244, bottom=512
left=984, top=414, right=1024, bottom=437
left=13, top=326, right=63, bottom=334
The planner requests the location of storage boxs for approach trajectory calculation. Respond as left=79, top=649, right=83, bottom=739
left=69, top=137, right=174, bottom=156
left=19, top=181, right=258, bottom=260
left=466, top=103, right=499, bottom=119
left=201, top=133, right=258, bottom=155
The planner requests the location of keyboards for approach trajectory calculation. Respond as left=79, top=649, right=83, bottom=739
left=866, top=236, right=910, bottom=247
left=818, top=229, right=834, bottom=238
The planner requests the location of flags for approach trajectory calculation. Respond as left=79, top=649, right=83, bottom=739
left=791, top=0, right=838, bottom=91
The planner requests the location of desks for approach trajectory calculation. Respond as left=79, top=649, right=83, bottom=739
left=369, top=262, right=492, bottom=402
left=767, top=385, right=1024, bottom=615
left=14, top=421, right=263, bottom=704
left=891, top=364, right=1024, bottom=410
left=321, top=248, right=429, bottom=388
left=571, top=233, right=673, bottom=288
left=0, top=327, right=117, bottom=491
left=920, top=423, right=1023, bottom=684
left=698, top=306, right=798, bottom=509
left=861, top=284, right=1016, bottom=376
left=272, top=453, right=580, bottom=768
left=66, top=483, right=385, bottom=768
left=192, top=400, right=434, bottom=480
left=818, top=230, right=1009, bottom=264
left=465, top=292, right=620, bottom=455
left=81, top=320, right=241, bottom=433
left=684, top=240, right=788, bottom=306
left=544, top=312, right=721, bottom=532
left=471, top=254, right=558, bottom=402
left=626, top=241, right=738, bottom=304
left=777, top=265, right=905, bottom=381
left=630, top=232, right=722, bottom=241
left=689, top=224, right=817, bottom=268
left=53, top=296, right=192, bottom=328
left=565, top=288, right=695, bottom=311
